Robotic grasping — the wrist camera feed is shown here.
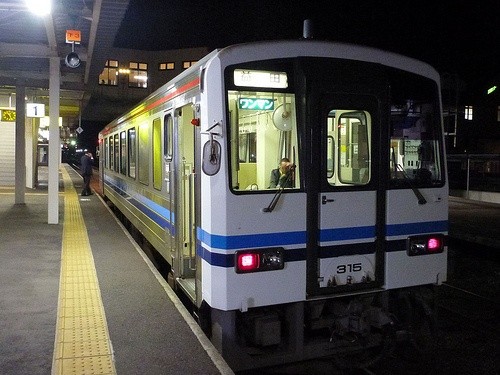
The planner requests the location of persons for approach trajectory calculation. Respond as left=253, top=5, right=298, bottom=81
left=417, top=135, right=435, bottom=172
left=267, top=158, right=294, bottom=189
left=80, top=150, right=94, bottom=196
left=61, top=142, right=70, bottom=163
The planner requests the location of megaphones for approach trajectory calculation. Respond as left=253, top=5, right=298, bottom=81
left=64, top=51, right=81, bottom=69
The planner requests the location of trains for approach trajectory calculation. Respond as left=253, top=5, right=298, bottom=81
left=98, top=18, right=453, bottom=375
